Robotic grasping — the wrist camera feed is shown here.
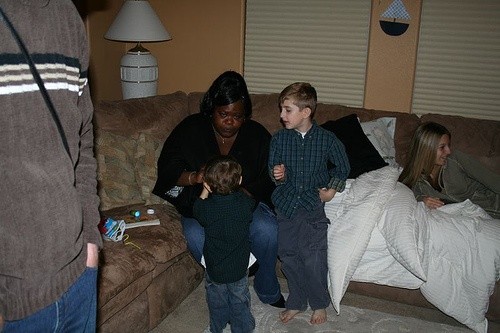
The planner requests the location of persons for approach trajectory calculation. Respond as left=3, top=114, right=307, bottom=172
left=192, top=158, right=258, bottom=333
left=0, top=0, right=102, bottom=333
left=153, top=71, right=286, bottom=308
left=268, top=82, right=350, bottom=324
left=398, top=122, right=500, bottom=219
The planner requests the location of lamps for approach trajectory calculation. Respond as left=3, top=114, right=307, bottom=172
left=104, top=0, right=173, bottom=100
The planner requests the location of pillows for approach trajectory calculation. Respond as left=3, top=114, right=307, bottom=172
left=316, top=114, right=405, bottom=193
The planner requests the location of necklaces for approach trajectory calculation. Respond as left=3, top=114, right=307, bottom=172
left=218, top=134, right=227, bottom=143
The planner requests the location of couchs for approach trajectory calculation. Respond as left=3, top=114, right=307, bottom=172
left=92, top=89, right=500, bottom=333
